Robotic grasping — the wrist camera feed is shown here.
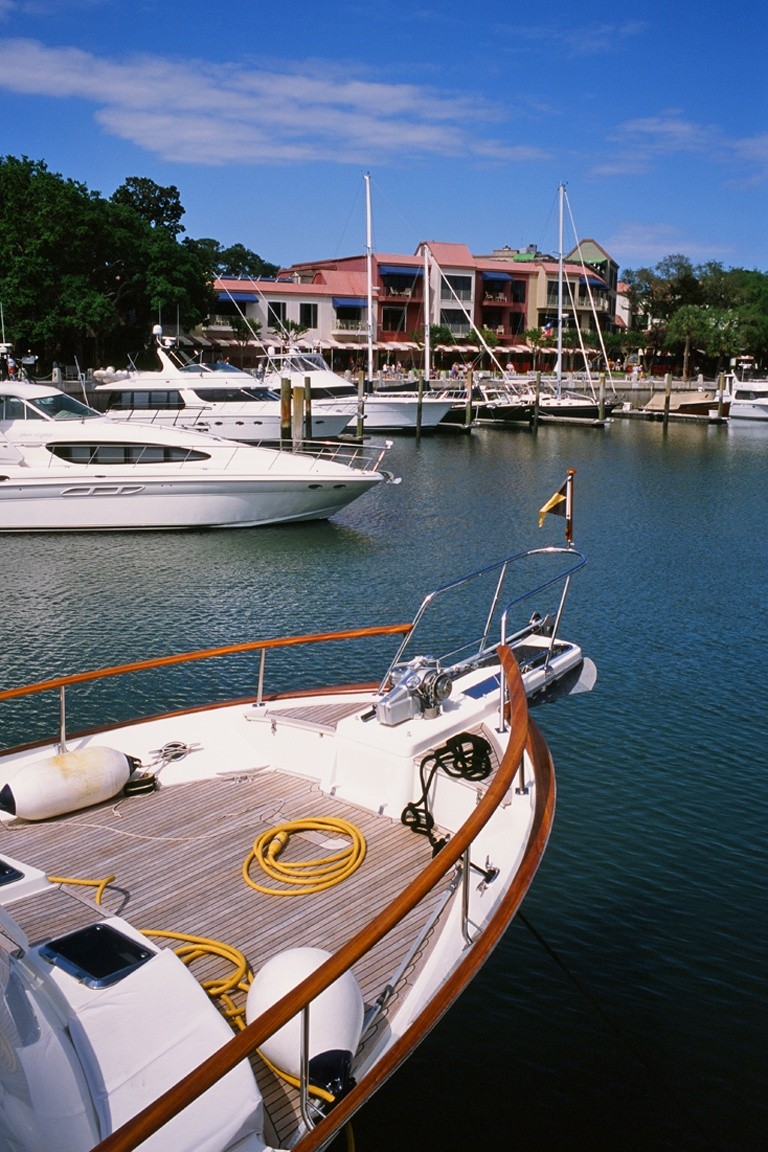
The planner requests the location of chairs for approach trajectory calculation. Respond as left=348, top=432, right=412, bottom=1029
left=484, top=323, right=505, bottom=335
left=485, top=291, right=504, bottom=302
left=213, top=315, right=229, bottom=326
left=336, top=318, right=365, bottom=330
left=389, top=286, right=412, bottom=298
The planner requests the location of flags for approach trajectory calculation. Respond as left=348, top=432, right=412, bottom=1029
left=538, top=477, right=573, bottom=541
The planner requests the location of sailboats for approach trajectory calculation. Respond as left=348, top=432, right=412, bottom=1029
left=210, top=172, right=469, bottom=429
left=493, top=180, right=625, bottom=421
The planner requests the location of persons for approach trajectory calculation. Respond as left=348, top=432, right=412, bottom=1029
left=0, top=348, right=39, bottom=383
left=349, top=356, right=405, bottom=379
left=588, top=359, right=645, bottom=381
left=217, top=356, right=229, bottom=365
left=429, top=361, right=514, bottom=380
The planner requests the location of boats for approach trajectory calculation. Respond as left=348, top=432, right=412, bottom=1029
left=0, top=299, right=403, bottom=532
left=91, top=325, right=370, bottom=441
left=708, top=370, right=768, bottom=419
left=377, top=242, right=541, bottom=422
left=0, top=468, right=600, bottom=1152
left=637, top=391, right=731, bottom=417
